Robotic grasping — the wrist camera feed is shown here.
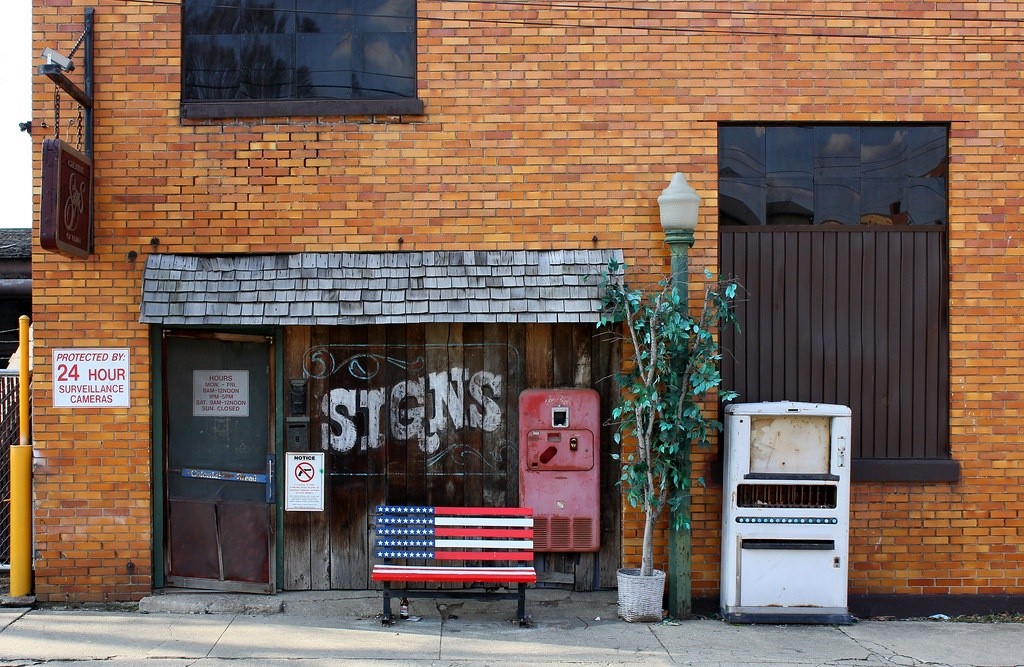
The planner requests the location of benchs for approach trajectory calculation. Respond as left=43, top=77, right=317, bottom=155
left=371, top=505, right=537, bottom=628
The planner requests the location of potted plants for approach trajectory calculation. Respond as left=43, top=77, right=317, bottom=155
left=586, top=255, right=741, bottom=622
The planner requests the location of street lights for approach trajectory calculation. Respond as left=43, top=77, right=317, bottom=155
left=657, top=171, right=702, bottom=620
